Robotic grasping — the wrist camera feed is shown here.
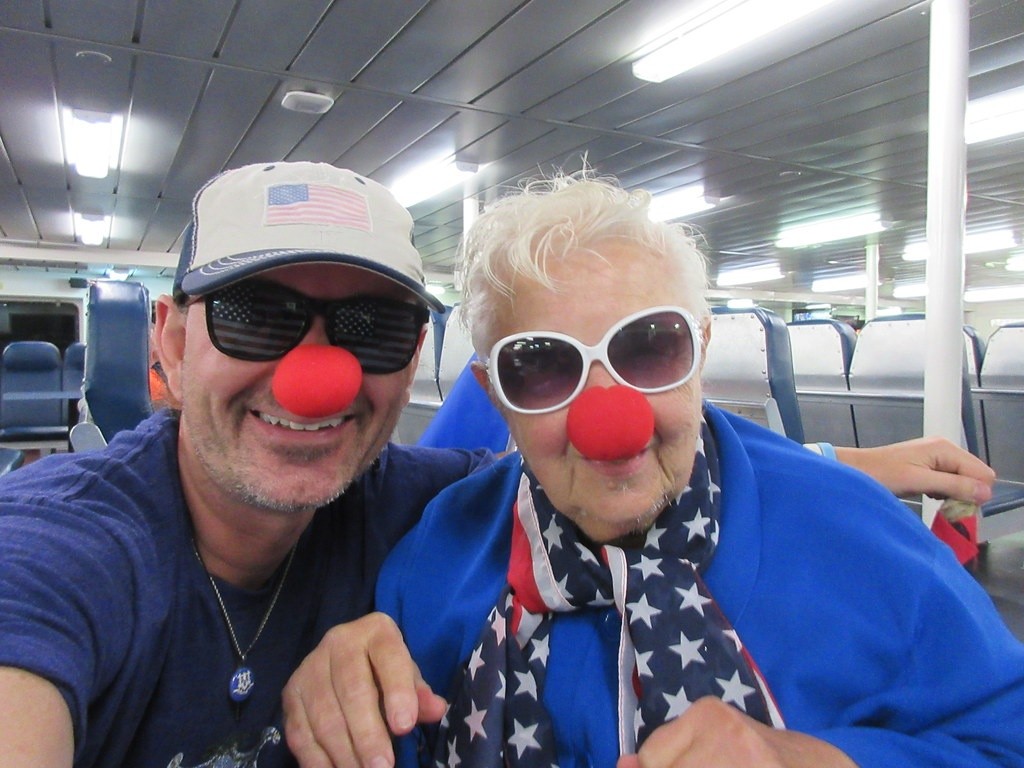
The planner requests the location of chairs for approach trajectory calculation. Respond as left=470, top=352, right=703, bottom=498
left=437, top=300, right=475, bottom=402
left=0, top=341, right=68, bottom=440
left=849, top=315, right=989, bottom=467
left=787, top=319, right=857, bottom=448
left=394, top=303, right=444, bottom=443
left=61, top=344, right=88, bottom=427
left=700, top=306, right=806, bottom=446
left=981, top=321, right=1024, bottom=482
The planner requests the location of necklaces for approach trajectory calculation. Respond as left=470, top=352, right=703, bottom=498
left=179, top=520, right=300, bottom=702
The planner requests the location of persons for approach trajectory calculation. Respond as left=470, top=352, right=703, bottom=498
left=283, top=147, right=1023, bottom=767
left=146, top=323, right=172, bottom=416
left=0, top=160, right=995, bottom=768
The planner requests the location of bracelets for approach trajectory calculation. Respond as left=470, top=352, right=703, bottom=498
left=802, top=442, right=838, bottom=468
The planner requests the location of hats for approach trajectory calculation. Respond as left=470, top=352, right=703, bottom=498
left=171, top=162, right=445, bottom=314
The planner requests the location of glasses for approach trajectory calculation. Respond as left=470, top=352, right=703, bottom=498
left=205, top=276, right=430, bottom=375
left=485, top=306, right=702, bottom=414
